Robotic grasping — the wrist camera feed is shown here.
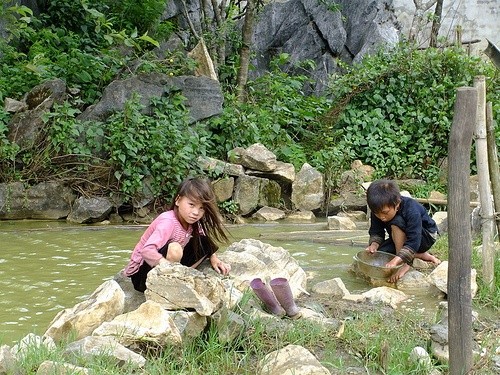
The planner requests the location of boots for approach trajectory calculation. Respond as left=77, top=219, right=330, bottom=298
left=249, top=278, right=287, bottom=319
left=270, top=277, right=301, bottom=319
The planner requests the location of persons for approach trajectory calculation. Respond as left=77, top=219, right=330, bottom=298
left=364, top=179, right=442, bottom=283
left=123, top=178, right=235, bottom=293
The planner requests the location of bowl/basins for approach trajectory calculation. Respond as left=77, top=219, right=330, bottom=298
left=353, top=250, right=404, bottom=279
